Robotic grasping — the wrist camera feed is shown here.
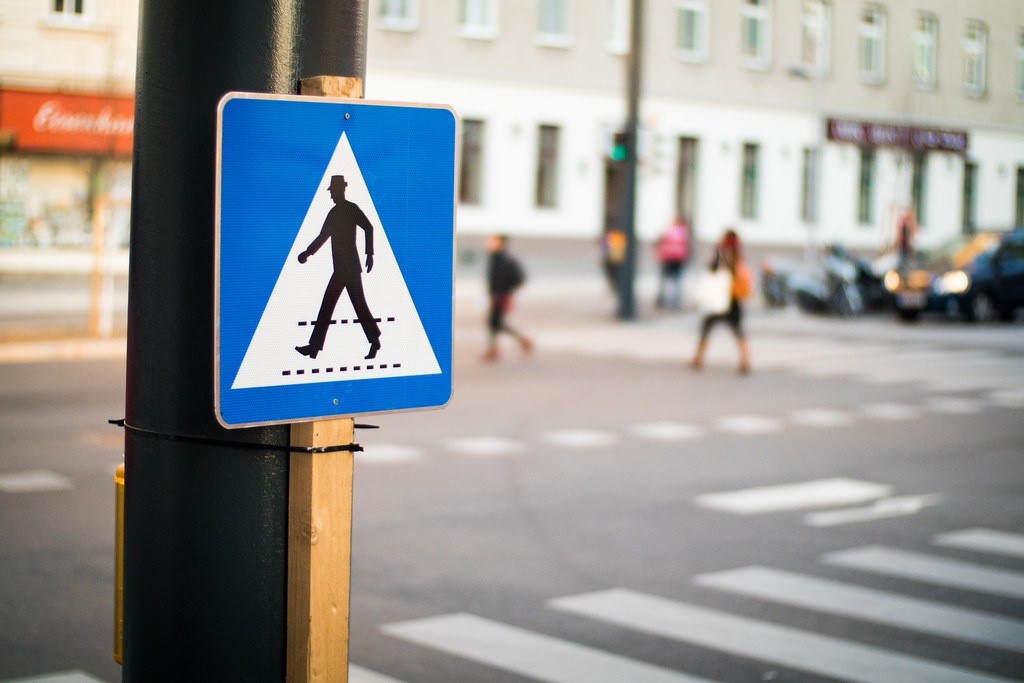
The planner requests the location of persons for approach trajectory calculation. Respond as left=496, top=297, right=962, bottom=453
left=598, top=215, right=691, bottom=319
left=688, top=230, right=751, bottom=372
left=479, top=233, right=533, bottom=361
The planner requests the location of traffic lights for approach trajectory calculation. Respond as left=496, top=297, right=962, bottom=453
left=610, top=133, right=629, bottom=167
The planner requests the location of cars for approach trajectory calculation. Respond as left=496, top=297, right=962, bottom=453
left=925, top=229, right=1024, bottom=323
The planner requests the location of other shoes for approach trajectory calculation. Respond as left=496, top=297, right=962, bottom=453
left=482, top=348, right=499, bottom=362
left=521, top=338, right=531, bottom=353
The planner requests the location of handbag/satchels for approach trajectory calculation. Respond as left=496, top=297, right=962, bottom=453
left=700, top=265, right=733, bottom=314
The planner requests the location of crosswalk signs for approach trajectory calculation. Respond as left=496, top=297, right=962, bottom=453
left=216, top=93, right=456, bottom=428
